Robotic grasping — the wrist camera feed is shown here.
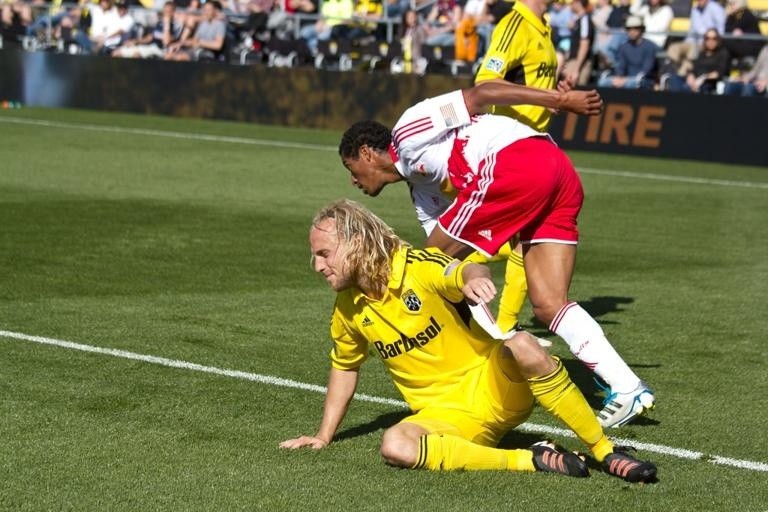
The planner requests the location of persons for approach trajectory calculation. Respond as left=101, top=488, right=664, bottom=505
left=461, top=1, right=578, bottom=347
left=274, top=199, right=662, bottom=487
left=0, top=0, right=768, bottom=100
left=336, top=77, right=658, bottom=432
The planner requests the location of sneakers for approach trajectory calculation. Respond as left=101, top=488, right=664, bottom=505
left=596, top=380, right=656, bottom=428
left=590, top=447, right=657, bottom=482
left=527, top=440, right=590, bottom=478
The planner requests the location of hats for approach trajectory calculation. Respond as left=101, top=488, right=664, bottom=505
left=625, top=15, right=644, bottom=28
